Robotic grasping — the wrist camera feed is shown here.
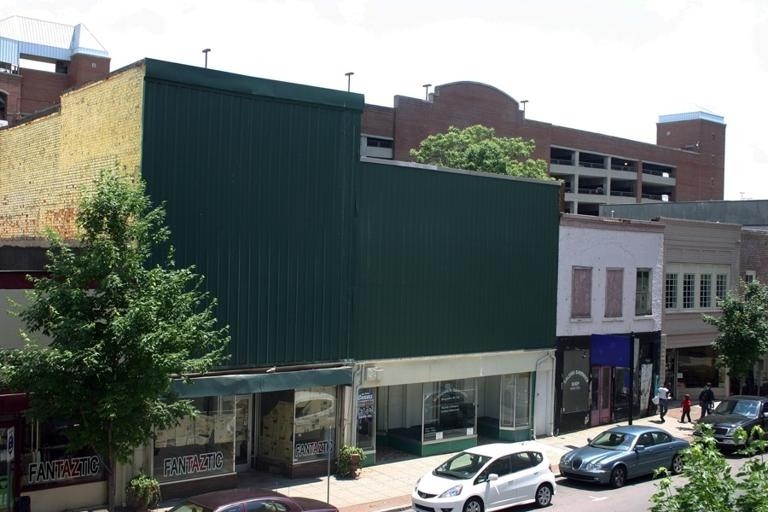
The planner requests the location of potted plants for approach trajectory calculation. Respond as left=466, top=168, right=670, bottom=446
left=333, top=445, right=366, bottom=480
left=125, top=471, right=162, bottom=512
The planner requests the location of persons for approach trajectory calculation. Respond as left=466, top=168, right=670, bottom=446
left=680, top=393, right=692, bottom=423
left=658, top=380, right=670, bottom=421
left=699, top=382, right=716, bottom=420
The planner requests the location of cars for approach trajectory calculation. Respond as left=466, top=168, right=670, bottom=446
left=559, top=426, right=690, bottom=487
left=411, top=443, right=557, bottom=512
left=166, top=489, right=339, bottom=512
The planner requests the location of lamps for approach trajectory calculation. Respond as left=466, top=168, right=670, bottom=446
left=266, top=366, right=277, bottom=373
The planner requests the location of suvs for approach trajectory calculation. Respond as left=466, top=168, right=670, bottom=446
left=693, top=395, right=768, bottom=453
